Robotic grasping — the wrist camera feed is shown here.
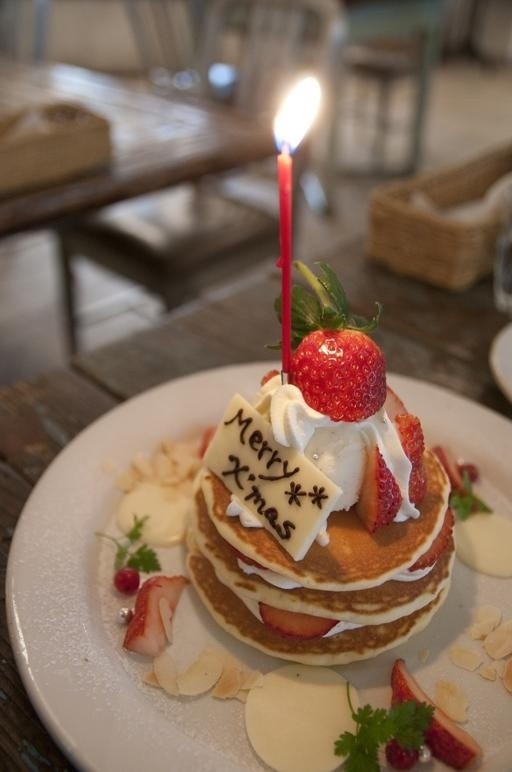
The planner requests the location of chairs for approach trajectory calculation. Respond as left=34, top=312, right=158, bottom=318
left=324, top=0, right=447, bottom=177
left=55, top=0, right=350, bottom=359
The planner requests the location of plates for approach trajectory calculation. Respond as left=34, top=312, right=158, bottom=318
left=7, top=359, right=512, bottom=772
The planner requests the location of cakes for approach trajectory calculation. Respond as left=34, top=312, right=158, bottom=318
left=184, top=260, right=457, bottom=671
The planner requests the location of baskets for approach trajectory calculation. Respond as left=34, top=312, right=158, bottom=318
left=368, top=141, right=511, bottom=291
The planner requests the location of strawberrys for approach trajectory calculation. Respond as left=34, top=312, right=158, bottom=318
left=125, top=571, right=187, bottom=660
left=388, top=658, right=482, bottom=771
